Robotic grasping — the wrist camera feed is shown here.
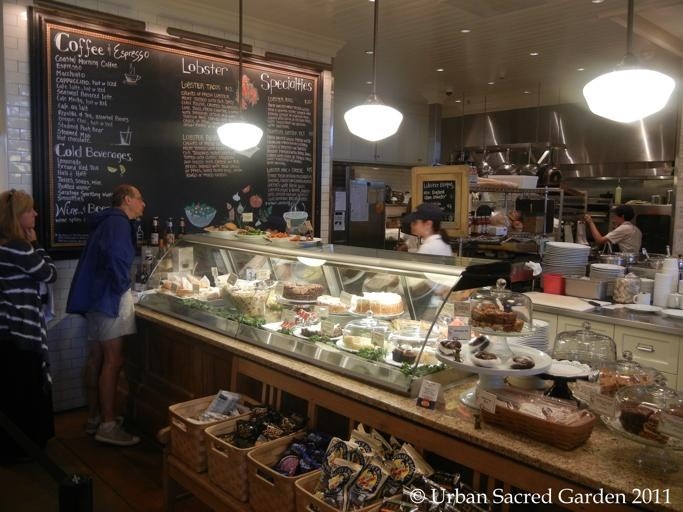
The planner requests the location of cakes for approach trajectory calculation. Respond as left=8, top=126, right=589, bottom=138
left=352, top=292, right=404, bottom=314
left=301, top=321, right=344, bottom=338
left=317, top=295, right=349, bottom=314
left=365, top=274, right=400, bottom=292
left=468, top=335, right=490, bottom=353
left=472, top=354, right=502, bottom=367
left=599, top=372, right=654, bottom=398
left=392, top=343, right=417, bottom=364
left=618, top=401, right=670, bottom=446
left=406, top=275, right=433, bottom=297
left=340, top=267, right=359, bottom=282
left=507, top=354, right=535, bottom=368
left=469, top=302, right=524, bottom=332
left=282, top=282, right=322, bottom=302
left=437, top=339, right=463, bottom=355
left=342, top=336, right=363, bottom=350
left=158, top=273, right=274, bottom=317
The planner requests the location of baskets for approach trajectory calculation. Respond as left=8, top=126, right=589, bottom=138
left=479, top=388, right=595, bottom=451
left=168, top=392, right=404, bottom=511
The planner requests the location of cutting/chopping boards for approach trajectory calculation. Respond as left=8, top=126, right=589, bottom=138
left=520, top=291, right=612, bottom=312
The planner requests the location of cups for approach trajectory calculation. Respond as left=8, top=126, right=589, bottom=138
left=120, top=131, right=132, bottom=145
left=653, top=259, right=683, bottom=308
left=124, top=73, right=141, bottom=83
left=632, top=292, right=651, bottom=305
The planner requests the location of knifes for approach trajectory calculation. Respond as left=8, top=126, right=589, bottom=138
left=577, top=297, right=601, bottom=306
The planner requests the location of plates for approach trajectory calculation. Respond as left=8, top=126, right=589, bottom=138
left=261, top=320, right=442, bottom=369
left=624, top=303, right=662, bottom=313
left=639, top=277, right=654, bottom=302
left=515, top=319, right=549, bottom=354
left=204, top=226, right=321, bottom=245
left=590, top=263, right=624, bottom=280
left=540, top=242, right=591, bottom=280
left=661, top=309, right=683, bottom=318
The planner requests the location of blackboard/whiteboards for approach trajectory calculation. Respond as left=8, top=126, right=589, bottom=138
left=28, top=5, right=322, bottom=261
left=411, top=165, right=469, bottom=237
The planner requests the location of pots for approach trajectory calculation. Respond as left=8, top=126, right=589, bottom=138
left=476, top=143, right=562, bottom=187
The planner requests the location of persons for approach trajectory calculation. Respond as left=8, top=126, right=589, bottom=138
left=0, top=188, right=58, bottom=469
left=66, top=183, right=145, bottom=448
left=266, top=216, right=287, bottom=234
left=583, top=205, right=642, bottom=253
left=392, top=202, right=453, bottom=308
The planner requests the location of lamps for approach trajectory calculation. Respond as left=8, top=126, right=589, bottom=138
left=583, top=0, right=676, bottom=125
left=217, top=0, right=263, bottom=152
left=344, top=0, right=404, bottom=142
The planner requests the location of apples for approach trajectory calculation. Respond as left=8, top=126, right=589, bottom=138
left=250, top=194, right=262, bottom=208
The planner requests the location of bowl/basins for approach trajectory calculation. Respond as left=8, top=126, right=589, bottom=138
left=184, top=206, right=217, bottom=228
left=283, top=211, right=308, bottom=229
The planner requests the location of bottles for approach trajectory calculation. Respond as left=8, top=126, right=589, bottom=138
left=615, top=183, right=622, bottom=206
left=468, top=211, right=491, bottom=235
left=135, top=216, right=185, bottom=284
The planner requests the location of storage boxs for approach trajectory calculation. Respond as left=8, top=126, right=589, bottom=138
left=168, top=392, right=266, bottom=475
left=247, top=431, right=329, bottom=512
left=295, top=471, right=404, bottom=511
left=204, top=412, right=302, bottom=504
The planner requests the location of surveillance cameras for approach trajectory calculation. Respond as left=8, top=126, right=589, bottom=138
left=446, top=89, right=453, bottom=96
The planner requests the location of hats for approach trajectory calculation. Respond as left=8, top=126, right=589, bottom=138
left=403, top=203, right=442, bottom=221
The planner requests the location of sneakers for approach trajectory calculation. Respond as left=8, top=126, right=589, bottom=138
left=94, top=422, right=141, bottom=446
left=85, top=415, right=124, bottom=435
